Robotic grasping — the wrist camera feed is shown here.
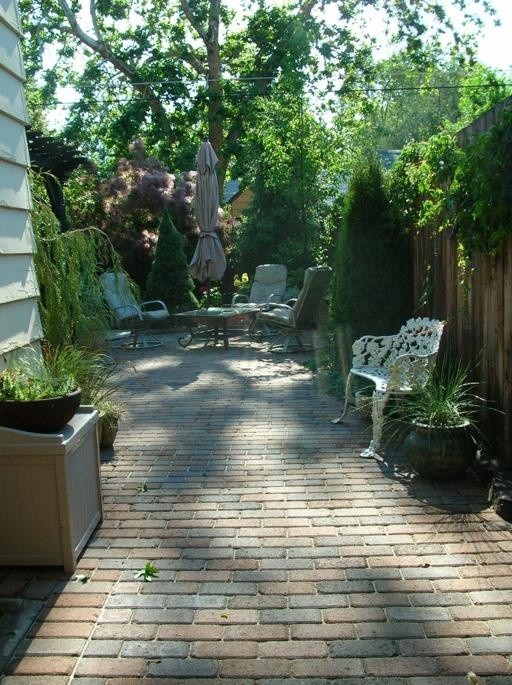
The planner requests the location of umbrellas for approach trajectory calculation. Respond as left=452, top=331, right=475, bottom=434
left=186, top=136, right=227, bottom=309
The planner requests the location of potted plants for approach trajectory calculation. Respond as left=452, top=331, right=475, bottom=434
left=88, top=395, right=128, bottom=448
left=341, top=348, right=508, bottom=482
left=0, top=364, right=82, bottom=434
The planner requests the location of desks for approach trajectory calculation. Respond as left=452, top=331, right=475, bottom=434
left=172, top=306, right=266, bottom=350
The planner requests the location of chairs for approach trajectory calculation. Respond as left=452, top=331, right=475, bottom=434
left=98, top=271, right=170, bottom=350
left=330, top=317, right=446, bottom=459
left=231, top=263, right=332, bottom=353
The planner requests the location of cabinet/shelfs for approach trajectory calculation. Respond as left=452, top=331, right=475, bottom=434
left=1, top=405, right=103, bottom=574
